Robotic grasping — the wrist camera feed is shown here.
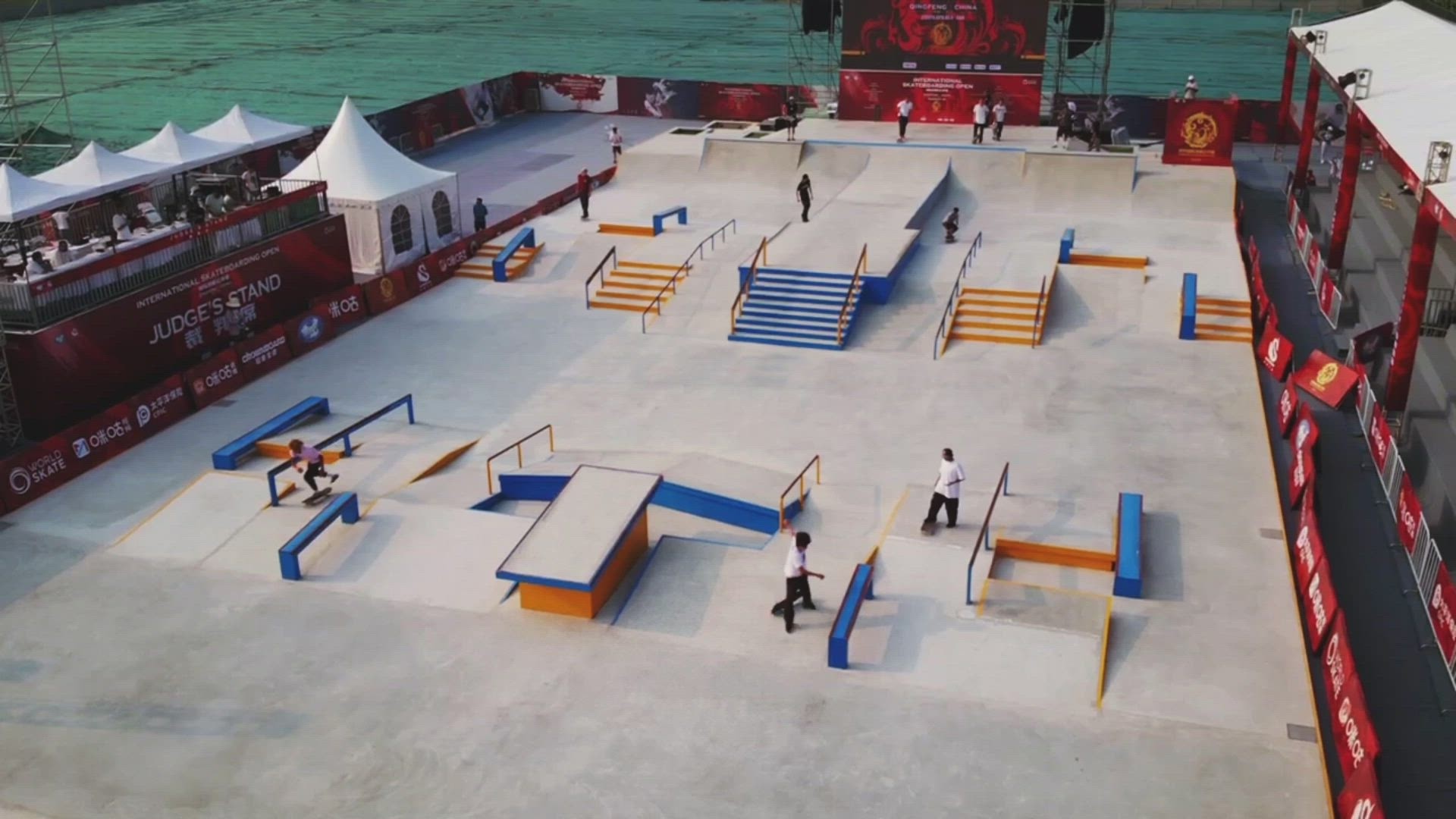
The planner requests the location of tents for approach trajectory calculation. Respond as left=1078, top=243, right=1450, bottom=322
left=257, top=96, right=464, bottom=275
left=182, top=104, right=321, bottom=201
left=32, top=140, right=177, bottom=247
left=0, top=162, right=82, bottom=280
left=115, top=120, right=256, bottom=214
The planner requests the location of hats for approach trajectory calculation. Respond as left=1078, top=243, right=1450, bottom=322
left=1188, top=75, right=1194, bottom=80
left=190, top=186, right=200, bottom=196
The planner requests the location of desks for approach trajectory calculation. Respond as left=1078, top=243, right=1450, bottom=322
left=0, top=207, right=266, bottom=312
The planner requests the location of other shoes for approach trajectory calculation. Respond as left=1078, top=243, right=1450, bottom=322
left=786, top=622, right=793, bottom=631
left=924, top=517, right=936, bottom=522
left=803, top=602, right=817, bottom=610
left=332, top=474, right=338, bottom=483
left=947, top=523, right=956, bottom=528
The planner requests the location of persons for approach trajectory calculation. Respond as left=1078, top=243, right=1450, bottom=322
left=1313, top=102, right=1347, bottom=165
left=781, top=96, right=798, bottom=141
left=287, top=439, right=340, bottom=495
left=241, top=161, right=262, bottom=204
left=771, top=518, right=825, bottom=632
left=973, top=89, right=1007, bottom=144
left=941, top=207, right=959, bottom=242
left=1052, top=97, right=1103, bottom=153
left=47, top=209, right=77, bottom=252
left=23, top=241, right=78, bottom=295
left=609, top=127, right=623, bottom=164
left=796, top=174, right=814, bottom=222
left=923, top=448, right=965, bottom=528
left=111, top=202, right=133, bottom=243
left=575, top=169, right=595, bottom=219
left=896, top=94, right=913, bottom=142
left=1183, top=75, right=1198, bottom=100
left=472, top=198, right=488, bottom=232
left=184, top=184, right=244, bottom=254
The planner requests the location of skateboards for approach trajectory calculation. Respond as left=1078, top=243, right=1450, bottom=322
left=921, top=516, right=936, bottom=535
left=773, top=591, right=806, bottom=615
left=946, top=236, right=955, bottom=242
left=302, top=487, right=332, bottom=505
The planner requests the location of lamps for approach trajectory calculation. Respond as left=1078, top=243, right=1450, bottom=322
left=1337, top=71, right=1357, bottom=89
left=1306, top=31, right=1315, bottom=43
